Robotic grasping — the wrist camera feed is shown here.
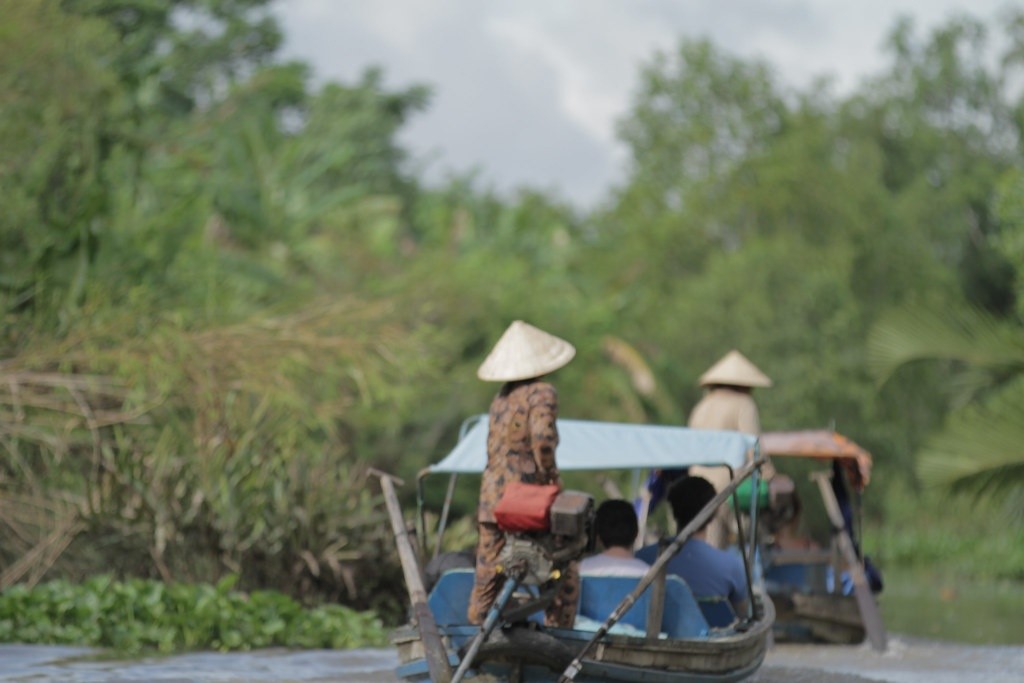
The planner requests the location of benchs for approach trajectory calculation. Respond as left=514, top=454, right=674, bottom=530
left=421, top=566, right=708, bottom=637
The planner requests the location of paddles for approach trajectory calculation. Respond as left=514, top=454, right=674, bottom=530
left=557, top=453, right=767, bottom=683
left=809, top=469, right=888, bottom=653
left=366, top=466, right=453, bottom=683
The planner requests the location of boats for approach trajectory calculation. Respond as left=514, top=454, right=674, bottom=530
left=630, top=428, right=883, bottom=646
left=366, top=408, right=772, bottom=682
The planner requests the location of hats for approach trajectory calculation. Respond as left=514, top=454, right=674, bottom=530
left=478, top=321, right=576, bottom=383
left=699, top=347, right=772, bottom=388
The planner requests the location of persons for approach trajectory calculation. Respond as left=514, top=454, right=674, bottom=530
left=758, top=457, right=800, bottom=551
left=424, top=503, right=479, bottom=592
left=576, top=498, right=652, bottom=578
left=635, top=475, right=749, bottom=627
left=686, top=349, right=775, bottom=546
left=642, top=465, right=690, bottom=546
left=467, top=319, right=576, bottom=630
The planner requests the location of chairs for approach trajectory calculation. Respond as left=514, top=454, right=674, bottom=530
left=771, top=559, right=838, bottom=595
left=695, top=593, right=740, bottom=636
